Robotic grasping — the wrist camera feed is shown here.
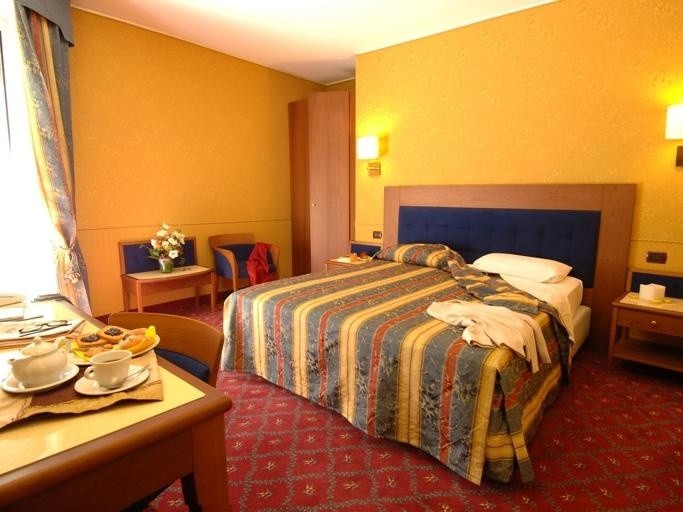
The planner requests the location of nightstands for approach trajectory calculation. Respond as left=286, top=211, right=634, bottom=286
left=608, top=269, right=683, bottom=383
left=324, top=240, right=382, bottom=270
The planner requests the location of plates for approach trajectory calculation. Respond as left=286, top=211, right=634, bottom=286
left=75, top=369, right=151, bottom=396
left=1, top=366, right=80, bottom=393
left=71, top=334, right=162, bottom=365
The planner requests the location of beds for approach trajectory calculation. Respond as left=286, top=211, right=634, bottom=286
left=224, top=184, right=634, bottom=482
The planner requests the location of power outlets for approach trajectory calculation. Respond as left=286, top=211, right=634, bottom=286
left=373, top=231, right=382, bottom=238
left=646, top=251, right=666, bottom=264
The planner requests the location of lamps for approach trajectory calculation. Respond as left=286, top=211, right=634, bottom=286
left=358, top=135, right=380, bottom=175
left=665, top=103, right=683, bottom=167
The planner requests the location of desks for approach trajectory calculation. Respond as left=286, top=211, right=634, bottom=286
left=118, top=237, right=216, bottom=315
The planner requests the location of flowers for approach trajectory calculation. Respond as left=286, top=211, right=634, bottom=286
left=139, top=222, right=185, bottom=260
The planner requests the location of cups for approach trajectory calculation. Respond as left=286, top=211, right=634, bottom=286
left=85, top=351, right=132, bottom=389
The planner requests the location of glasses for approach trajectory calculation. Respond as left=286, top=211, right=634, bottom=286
left=18, top=320, right=72, bottom=338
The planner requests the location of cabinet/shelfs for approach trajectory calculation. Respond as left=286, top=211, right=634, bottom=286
left=288, top=90, right=356, bottom=274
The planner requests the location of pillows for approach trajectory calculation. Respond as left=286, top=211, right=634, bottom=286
left=377, top=244, right=458, bottom=268
left=471, top=253, right=571, bottom=283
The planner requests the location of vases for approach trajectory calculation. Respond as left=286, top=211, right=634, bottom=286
left=159, top=259, right=173, bottom=272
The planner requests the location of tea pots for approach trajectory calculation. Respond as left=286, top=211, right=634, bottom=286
left=8, top=336, right=73, bottom=387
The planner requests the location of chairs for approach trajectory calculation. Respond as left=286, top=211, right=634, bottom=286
left=210, top=234, right=280, bottom=309
left=107, top=312, right=224, bottom=507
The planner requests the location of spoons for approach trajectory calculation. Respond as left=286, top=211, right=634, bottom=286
left=106, top=364, right=155, bottom=390
